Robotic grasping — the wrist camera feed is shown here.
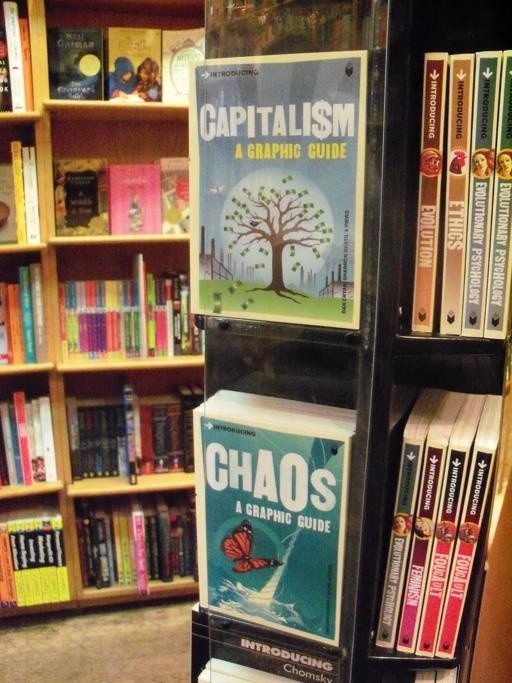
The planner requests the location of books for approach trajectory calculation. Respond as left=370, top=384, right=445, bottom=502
left=374, top=50, right=512, bottom=683
left=2, top=27, right=198, bottom=605
left=191, top=389, right=357, bottom=683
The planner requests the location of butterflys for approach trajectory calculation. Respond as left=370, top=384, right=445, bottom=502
left=220, top=518, right=284, bottom=574
